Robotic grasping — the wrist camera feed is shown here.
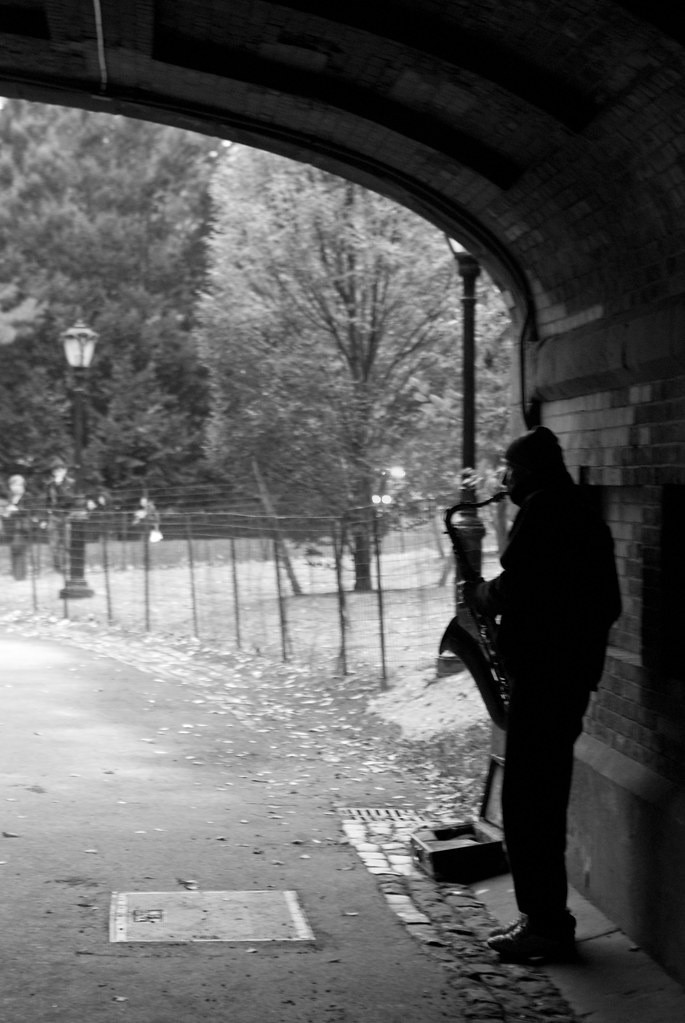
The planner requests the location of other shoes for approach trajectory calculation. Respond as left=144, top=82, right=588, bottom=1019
left=488, top=912, right=577, bottom=958
left=488, top=907, right=575, bottom=936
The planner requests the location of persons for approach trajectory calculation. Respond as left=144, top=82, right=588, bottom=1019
left=139, top=497, right=159, bottom=540
left=41, top=460, right=75, bottom=571
left=0, top=474, right=34, bottom=579
left=464, top=427, right=620, bottom=953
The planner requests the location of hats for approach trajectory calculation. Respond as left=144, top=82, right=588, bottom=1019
left=505, top=427, right=561, bottom=477
left=47, top=458, right=66, bottom=469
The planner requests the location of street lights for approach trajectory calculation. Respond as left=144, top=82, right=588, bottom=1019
left=437, top=235, right=486, bottom=677
left=58, top=314, right=101, bottom=598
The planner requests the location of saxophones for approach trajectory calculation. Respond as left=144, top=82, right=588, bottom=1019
left=438, top=490, right=527, bottom=730
